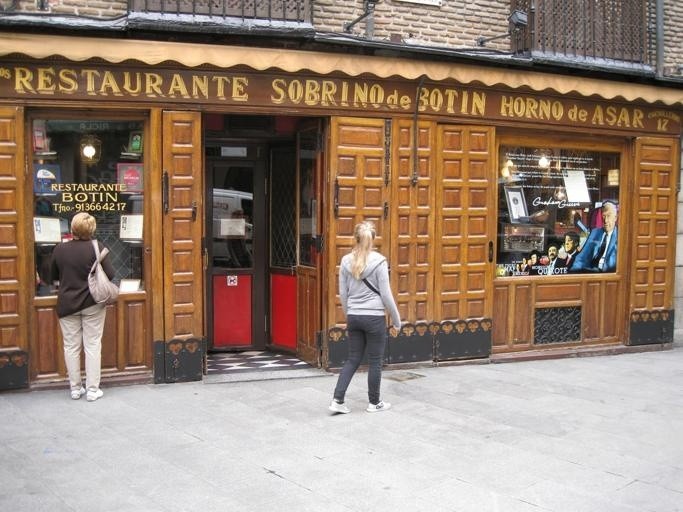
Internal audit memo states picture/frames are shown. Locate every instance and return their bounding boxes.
[127,130,143,154]
[503,186,529,223]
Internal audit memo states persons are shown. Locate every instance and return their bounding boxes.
[47,213,114,401]
[571,199,617,273]
[225,207,251,269]
[514,246,563,274]
[328,221,401,415]
[565,229,579,268]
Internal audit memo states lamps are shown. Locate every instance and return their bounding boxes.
[78,120,102,167]
[477,10,528,46]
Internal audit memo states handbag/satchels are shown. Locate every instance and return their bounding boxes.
[88,263,119,306]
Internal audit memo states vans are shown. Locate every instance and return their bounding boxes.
[210,188,253,267]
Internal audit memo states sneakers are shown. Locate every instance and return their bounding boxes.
[71,387,85,400]
[366,400,392,412]
[328,401,351,413]
[87,389,103,402]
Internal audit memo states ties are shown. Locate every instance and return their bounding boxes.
[591,233,608,268]
